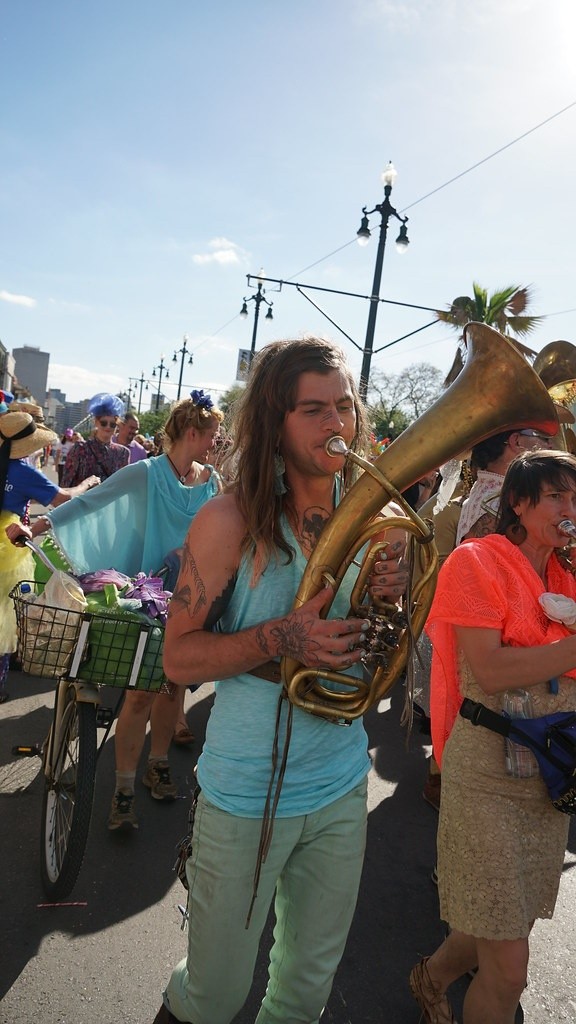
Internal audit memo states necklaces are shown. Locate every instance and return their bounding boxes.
[167,453,191,485]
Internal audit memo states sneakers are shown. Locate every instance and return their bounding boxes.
[142,757,180,800]
[106,786,139,831]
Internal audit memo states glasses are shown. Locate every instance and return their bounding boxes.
[96,418,119,429]
[519,428,556,444]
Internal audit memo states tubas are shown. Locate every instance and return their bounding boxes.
[281,321,574,728]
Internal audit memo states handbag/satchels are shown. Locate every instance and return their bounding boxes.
[460,699,575,817]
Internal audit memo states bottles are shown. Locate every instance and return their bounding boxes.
[501,687,539,777]
[15,584,37,626]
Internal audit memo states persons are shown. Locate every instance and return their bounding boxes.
[172,425,235,743]
[5,387,226,832]
[161,335,409,1024]
[406,450,576,1024]
[0,390,165,702]
[399,426,558,883]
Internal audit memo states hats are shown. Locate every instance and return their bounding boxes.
[7,401,53,431]
[0,411,58,459]
[66,428,74,437]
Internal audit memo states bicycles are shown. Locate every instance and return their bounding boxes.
[8,535,175,902]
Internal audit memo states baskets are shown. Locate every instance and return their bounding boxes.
[8,577,178,693]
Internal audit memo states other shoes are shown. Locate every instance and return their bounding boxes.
[423,777,440,809]
[419,718,433,735]
[172,727,195,743]
[409,956,457,1024]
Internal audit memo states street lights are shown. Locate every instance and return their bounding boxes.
[122,381,136,414]
[354,159,411,404]
[173,333,197,405]
[133,367,148,415]
[388,420,395,437]
[239,267,274,386]
[150,350,170,418]
